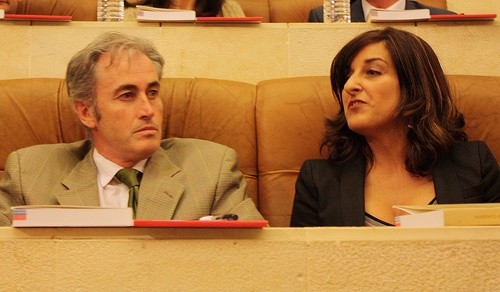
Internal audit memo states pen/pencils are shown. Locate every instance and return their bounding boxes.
[192,213,239,221]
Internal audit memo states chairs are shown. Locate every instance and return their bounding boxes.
[254,74,500,228]
[0,77,257,227]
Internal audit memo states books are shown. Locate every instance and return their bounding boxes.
[8,203,135,229]
[392,202,499,227]
[136,5,199,23]
[365,8,431,23]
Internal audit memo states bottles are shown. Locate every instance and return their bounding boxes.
[97,0,124,21]
[323,0,350,23]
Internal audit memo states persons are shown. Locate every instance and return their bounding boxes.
[0,30,268,227]
[121,0,245,23]
[0,0,98,22]
[307,1,456,23]
[289,29,500,228]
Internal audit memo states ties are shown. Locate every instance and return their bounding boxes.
[116,168,140,220]
